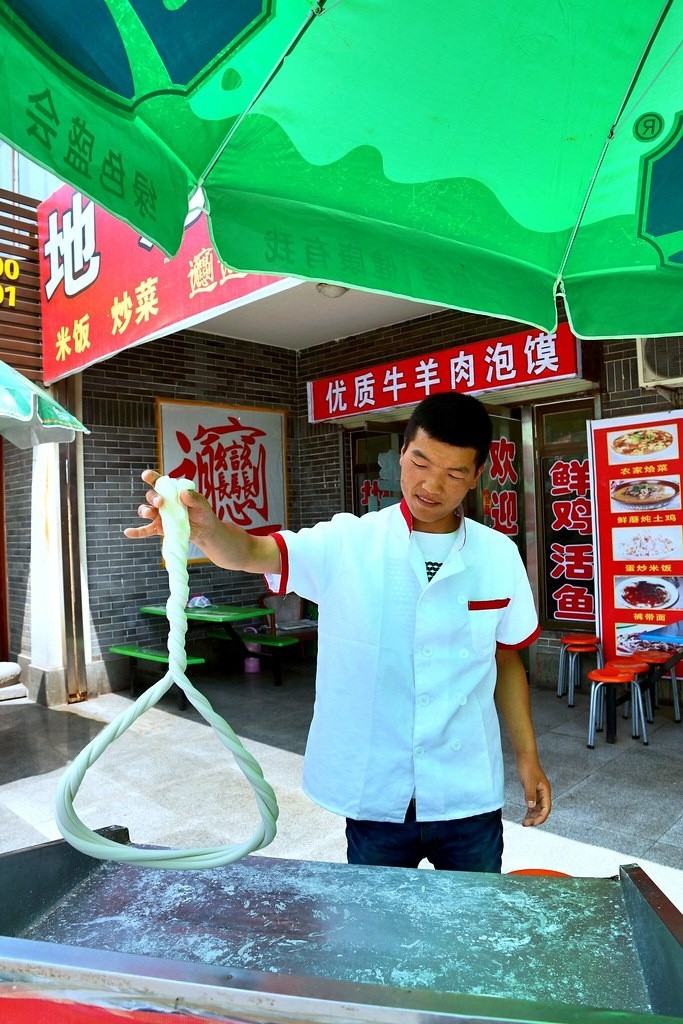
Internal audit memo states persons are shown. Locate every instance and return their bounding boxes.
[120,392,552,874]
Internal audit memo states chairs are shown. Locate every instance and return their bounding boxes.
[257,592,317,674]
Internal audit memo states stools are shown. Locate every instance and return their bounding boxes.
[622,651,682,724]
[562,645,600,697]
[557,634,604,700]
[586,668,648,751]
[596,658,649,740]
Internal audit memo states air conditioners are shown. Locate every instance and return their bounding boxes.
[635,336,683,392]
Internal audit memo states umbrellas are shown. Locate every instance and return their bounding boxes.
[0,358,94,451]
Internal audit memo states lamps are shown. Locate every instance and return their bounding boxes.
[316,283,350,298]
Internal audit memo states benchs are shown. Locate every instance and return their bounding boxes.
[204,629,299,687]
[109,644,205,712]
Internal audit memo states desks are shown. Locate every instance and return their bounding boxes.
[139,605,275,672]
